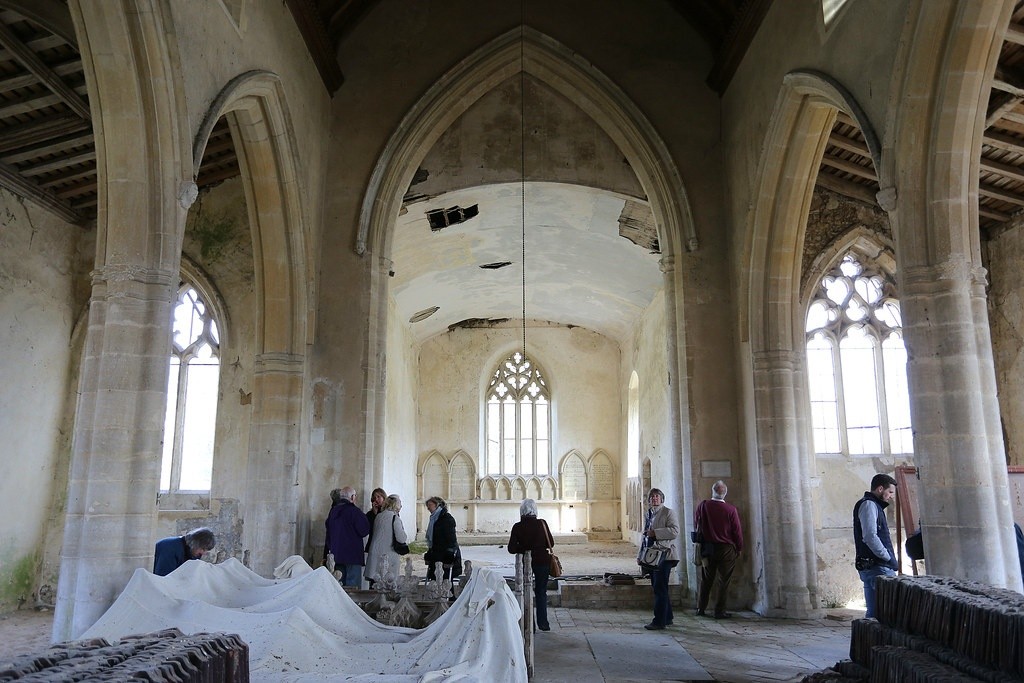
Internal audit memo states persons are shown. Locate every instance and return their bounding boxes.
[694,480,744,619]
[853,474,898,618]
[637,488,680,630]
[508,499,555,634]
[153,529,216,577]
[424,497,462,601]
[323,487,407,590]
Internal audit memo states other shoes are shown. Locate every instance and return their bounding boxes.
[697,608,704,615]
[715,613,731,619]
[644,624,665,630]
[665,620,673,624]
[539,624,550,631]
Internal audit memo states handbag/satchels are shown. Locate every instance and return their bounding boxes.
[639,547,666,569]
[548,553,562,577]
[691,515,702,566]
[392,515,410,555]
